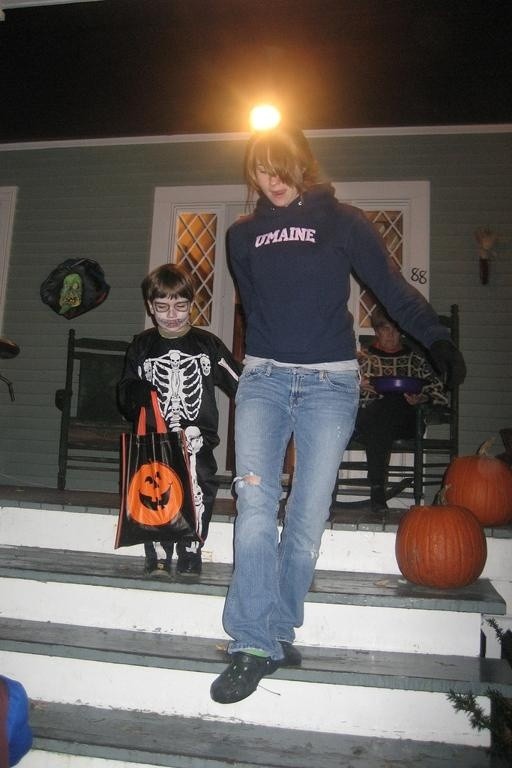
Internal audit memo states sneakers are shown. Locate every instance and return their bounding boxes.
[144,557,171,579]
[370,485,388,513]
[176,556,202,576]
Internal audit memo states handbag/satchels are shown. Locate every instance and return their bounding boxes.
[112,389,202,548]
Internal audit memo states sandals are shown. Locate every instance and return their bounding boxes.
[278,640,302,666]
[209,650,278,705]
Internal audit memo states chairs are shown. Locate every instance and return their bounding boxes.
[54,329,140,495]
[335,303,460,509]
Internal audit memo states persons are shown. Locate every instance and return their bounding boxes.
[355,301,449,512]
[116,264,244,574]
[210,122,466,704]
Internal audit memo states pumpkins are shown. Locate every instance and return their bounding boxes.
[394,483,488,589]
[443,435,512,527]
